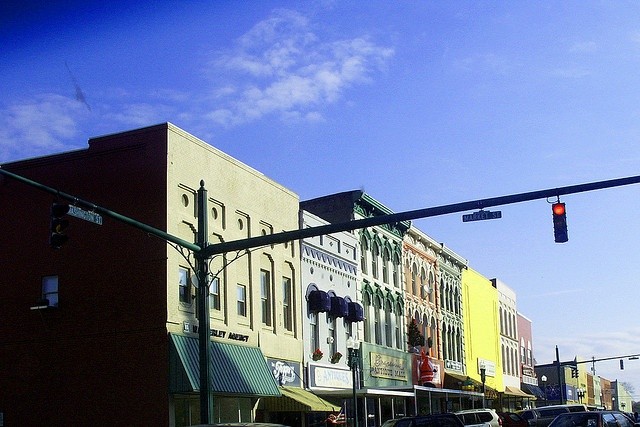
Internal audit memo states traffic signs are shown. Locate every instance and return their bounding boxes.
[463,210,502,221]
[66,202,102,225]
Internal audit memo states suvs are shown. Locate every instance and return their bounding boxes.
[451,407,502,426]
[379,413,466,426]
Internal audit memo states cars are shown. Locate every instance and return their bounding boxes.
[547,410,640,426]
[498,411,528,426]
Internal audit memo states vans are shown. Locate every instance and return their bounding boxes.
[517,404,590,426]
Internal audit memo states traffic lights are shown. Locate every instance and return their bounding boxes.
[575,369,579,378]
[571,368,575,378]
[552,202,568,242]
[49,201,70,247]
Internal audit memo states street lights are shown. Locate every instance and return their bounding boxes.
[621,401,627,410]
[480,362,486,393]
[600,392,603,406]
[345,335,361,427]
[612,397,615,407]
[577,388,586,403]
[542,374,548,405]
